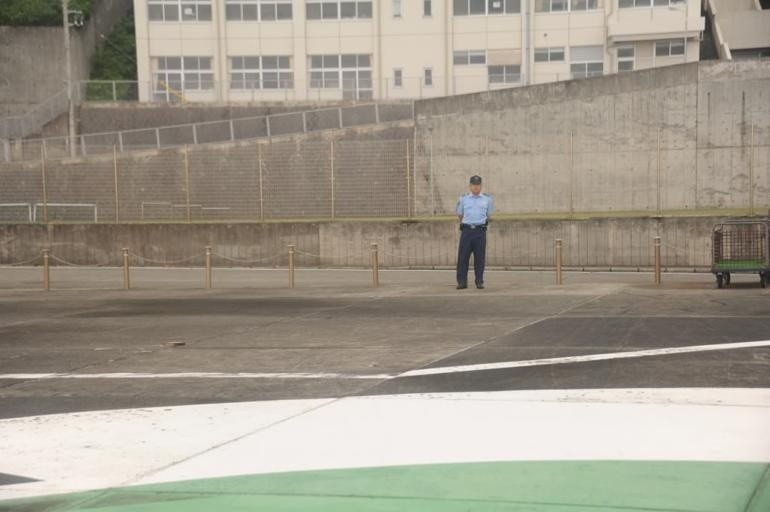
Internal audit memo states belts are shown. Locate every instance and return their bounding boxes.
[462,225,486,230]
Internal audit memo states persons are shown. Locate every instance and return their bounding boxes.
[451,173,494,292]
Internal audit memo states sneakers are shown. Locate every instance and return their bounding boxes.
[475,279,485,288]
[457,282,468,289]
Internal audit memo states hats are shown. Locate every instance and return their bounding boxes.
[470,176,481,184]
